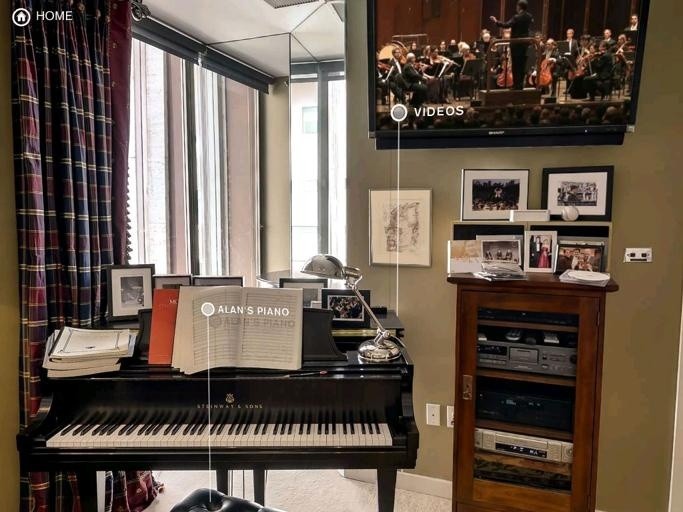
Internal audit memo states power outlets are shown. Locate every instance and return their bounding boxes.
[446,405,454,428]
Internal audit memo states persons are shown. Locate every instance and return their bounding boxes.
[531,236,550,268]
[486,249,512,261]
[557,182,597,206]
[375,0,638,110]
[559,248,599,272]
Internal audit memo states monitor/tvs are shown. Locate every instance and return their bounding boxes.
[366,0,652,151]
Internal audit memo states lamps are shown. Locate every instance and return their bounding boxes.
[299,253,406,363]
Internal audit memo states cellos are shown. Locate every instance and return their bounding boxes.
[497,50,513,87]
[535,42,556,87]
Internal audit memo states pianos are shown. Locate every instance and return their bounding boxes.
[15,306,417,511]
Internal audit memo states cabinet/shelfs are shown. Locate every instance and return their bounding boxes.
[446,273,618,512]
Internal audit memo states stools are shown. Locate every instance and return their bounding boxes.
[168,487,287,512]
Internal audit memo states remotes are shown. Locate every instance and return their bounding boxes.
[478,333,488,341]
[505,329,522,341]
[543,333,560,344]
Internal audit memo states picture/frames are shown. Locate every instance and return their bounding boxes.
[105,264,155,322]
[459,168,529,221]
[475,234,523,266]
[368,188,431,269]
[541,165,614,221]
[523,230,557,273]
[558,244,604,275]
[321,289,370,329]
[152,276,190,288]
[279,278,327,309]
[192,277,242,286]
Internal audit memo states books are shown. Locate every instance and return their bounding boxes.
[43,325,135,379]
[148,285,304,375]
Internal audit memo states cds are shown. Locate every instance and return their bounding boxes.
[568,270,610,281]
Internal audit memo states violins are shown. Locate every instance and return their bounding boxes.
[584,50,605,62]
[378,53,477,72]
